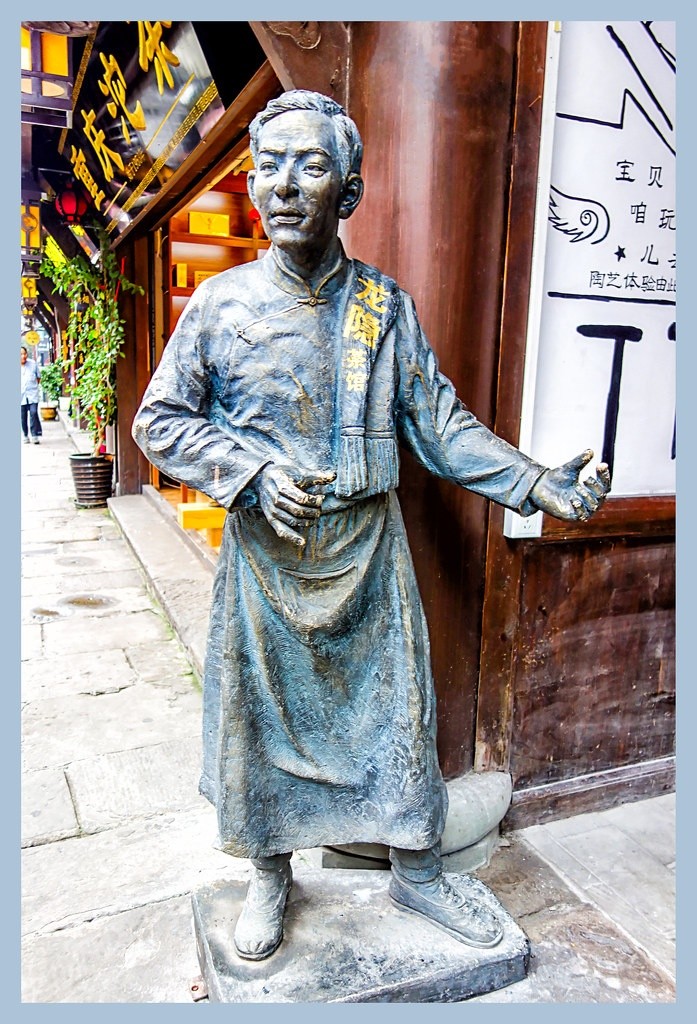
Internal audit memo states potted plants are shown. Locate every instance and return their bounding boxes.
[38,219,147,504]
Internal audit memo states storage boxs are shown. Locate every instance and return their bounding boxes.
[188,212,230,236]
[173,263,188,288]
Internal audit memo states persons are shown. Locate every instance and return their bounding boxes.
[132,89,610,958]
[21,346,44,444]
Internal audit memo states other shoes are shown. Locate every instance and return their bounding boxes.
[33,437,39,444]
[25,437,31,444]
